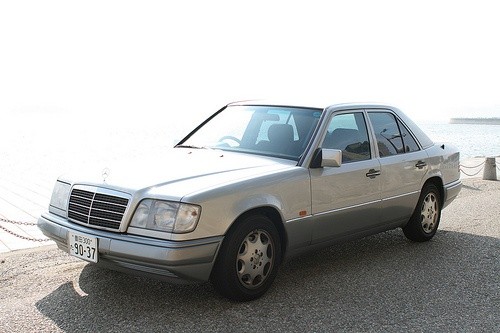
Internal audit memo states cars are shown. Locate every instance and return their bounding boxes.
[37,96,462,301]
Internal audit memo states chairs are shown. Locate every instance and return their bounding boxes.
[256,123,301,158]
